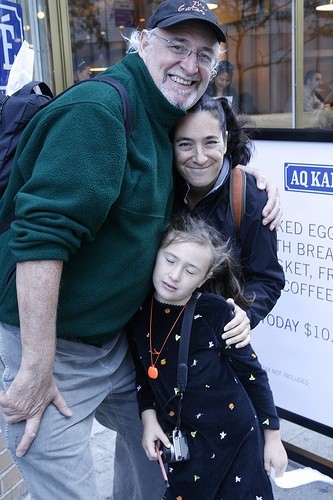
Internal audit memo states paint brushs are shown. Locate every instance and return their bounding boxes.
[154,443,171,489]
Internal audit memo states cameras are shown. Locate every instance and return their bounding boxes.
[161,430,190,462]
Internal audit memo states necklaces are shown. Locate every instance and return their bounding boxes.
[148,298,186,380]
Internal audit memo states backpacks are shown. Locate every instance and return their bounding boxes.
[0,76,132,235]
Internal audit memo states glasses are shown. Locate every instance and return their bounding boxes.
[150,32,217,69]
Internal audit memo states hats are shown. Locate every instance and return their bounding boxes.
[147,0,226,44]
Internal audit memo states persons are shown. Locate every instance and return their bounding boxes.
[283,70,325,111]
[205,60,238,112]
[172,93,286,348]
[127,215,288,500]
[72,56,90,84]
[0,0,282,500]
